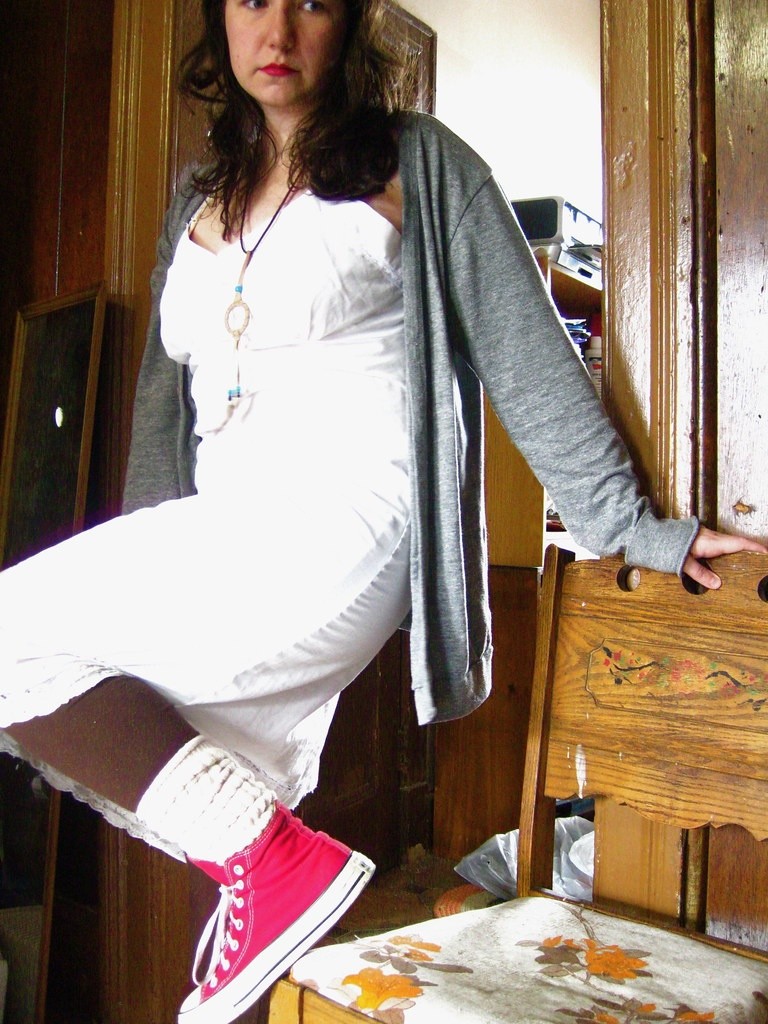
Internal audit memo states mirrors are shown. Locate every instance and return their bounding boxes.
[0,279,108,1024]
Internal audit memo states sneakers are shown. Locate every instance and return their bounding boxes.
[179,799,377,1024]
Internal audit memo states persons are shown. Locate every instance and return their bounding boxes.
[0,1,767,1024]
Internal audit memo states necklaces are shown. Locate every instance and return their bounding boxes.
[225,158,305,400]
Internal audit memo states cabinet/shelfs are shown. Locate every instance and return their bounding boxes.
[483,254,603,567]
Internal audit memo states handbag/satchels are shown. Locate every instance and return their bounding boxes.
[454,814,594,903]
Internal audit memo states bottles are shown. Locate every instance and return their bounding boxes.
[583,336,602,394]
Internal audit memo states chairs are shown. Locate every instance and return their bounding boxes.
[255,544,768,1024]
